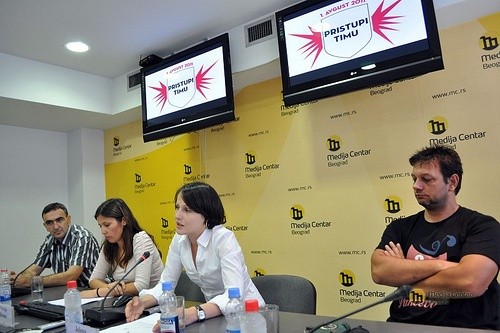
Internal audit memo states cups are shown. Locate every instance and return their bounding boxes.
[259,303,280,333]
[31,276,43,303]
[175,296,186,332]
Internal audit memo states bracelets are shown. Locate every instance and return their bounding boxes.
[96,288,100,297]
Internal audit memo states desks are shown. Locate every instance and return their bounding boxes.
[0,286,500,333]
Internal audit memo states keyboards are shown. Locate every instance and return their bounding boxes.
[17,300,65,321]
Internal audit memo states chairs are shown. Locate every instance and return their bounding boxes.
[250,276,316,315]
[174,271,206,303]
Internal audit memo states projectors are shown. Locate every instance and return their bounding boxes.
[139,54,164,67]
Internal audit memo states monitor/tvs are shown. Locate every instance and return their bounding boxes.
[140,30,236,143]
[275,0,444,107]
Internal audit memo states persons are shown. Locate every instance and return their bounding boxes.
[371,146,500,331]
[125,182,266,333]
[7,202,100,289]
[79,198,164,298]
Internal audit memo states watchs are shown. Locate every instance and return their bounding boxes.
[194,304,206,322]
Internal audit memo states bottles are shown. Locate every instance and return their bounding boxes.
[240,299,267,333]
[224,287,244,333]
[158,282,179,333]
[0,267,12,305]
[64,281,83,333]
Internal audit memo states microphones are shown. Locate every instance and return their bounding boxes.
[9,248,53,298]
[86,250,151,325]
[304,284,413,333]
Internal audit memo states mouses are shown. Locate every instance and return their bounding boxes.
[112,292,134,307]
